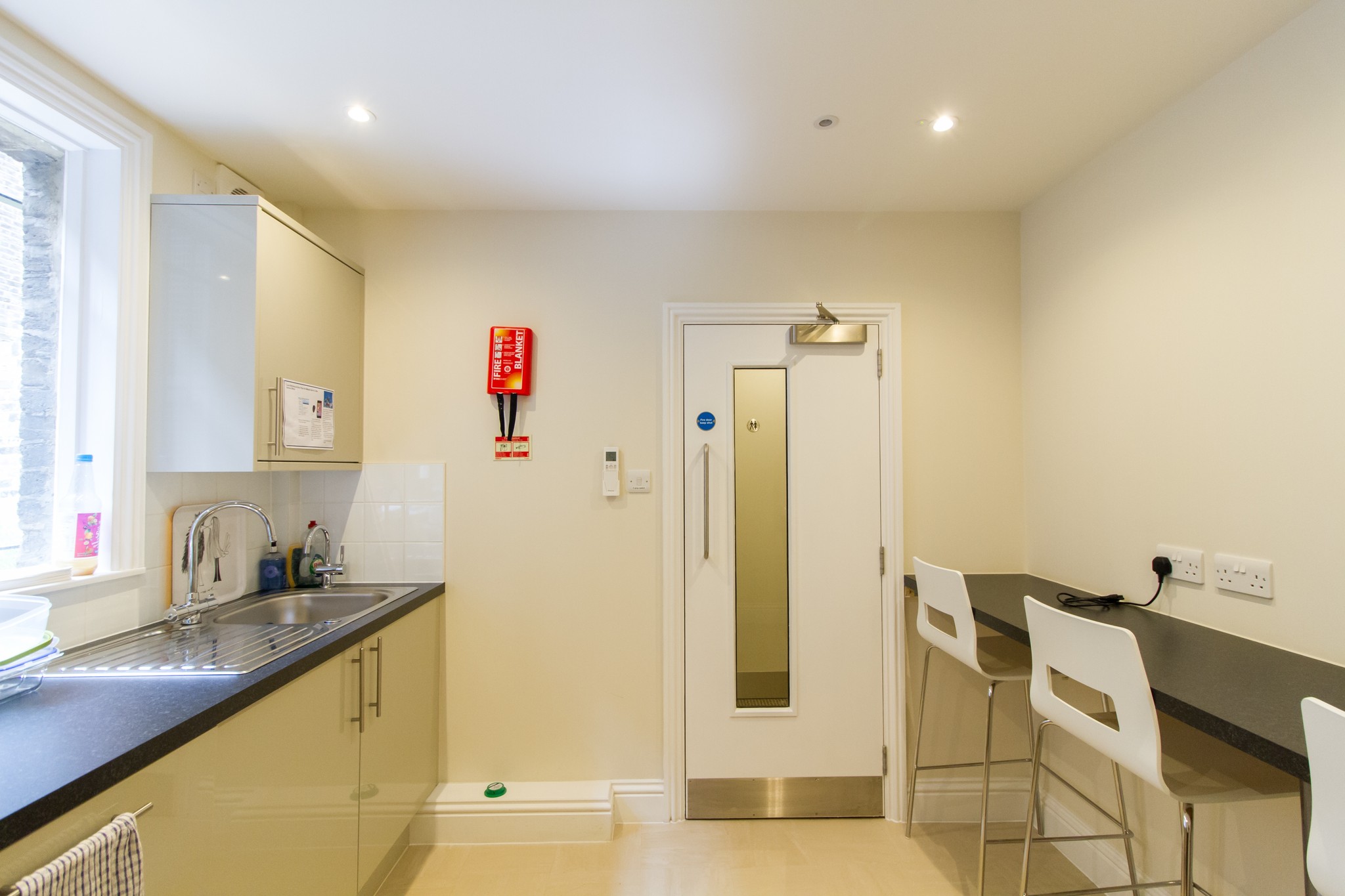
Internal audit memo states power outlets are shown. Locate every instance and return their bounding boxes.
[1157,544,1205,585]
[1214,555,1274,599]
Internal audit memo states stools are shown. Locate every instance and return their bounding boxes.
[906,556,1138,896]
[1021,594,1302,896]
[1301,697,1345,896]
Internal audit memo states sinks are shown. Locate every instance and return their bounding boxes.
[210,583,418,625]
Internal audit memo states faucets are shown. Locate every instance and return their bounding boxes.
[302,524,346,588]
[163,499,279,629]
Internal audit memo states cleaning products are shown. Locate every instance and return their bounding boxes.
[259,541,287,589]
[298,520,322,588]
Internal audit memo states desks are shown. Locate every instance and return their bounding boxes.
[904,573,1344,784]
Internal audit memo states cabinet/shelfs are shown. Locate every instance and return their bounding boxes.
[219,595,443,896]
[149,195,365,473]
